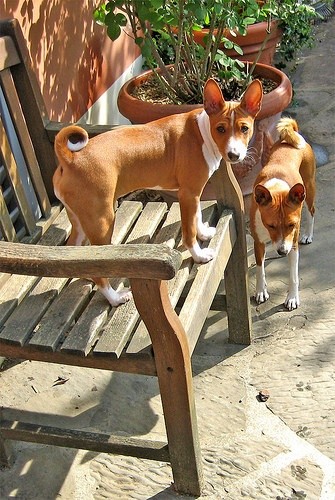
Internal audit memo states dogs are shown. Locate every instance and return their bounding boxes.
[52,77,264,308]
[251,116,314,311]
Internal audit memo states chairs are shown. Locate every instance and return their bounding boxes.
[0,17,251,497]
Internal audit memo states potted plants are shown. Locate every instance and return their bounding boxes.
[163,0,303,107]
[91,0,324,222]
